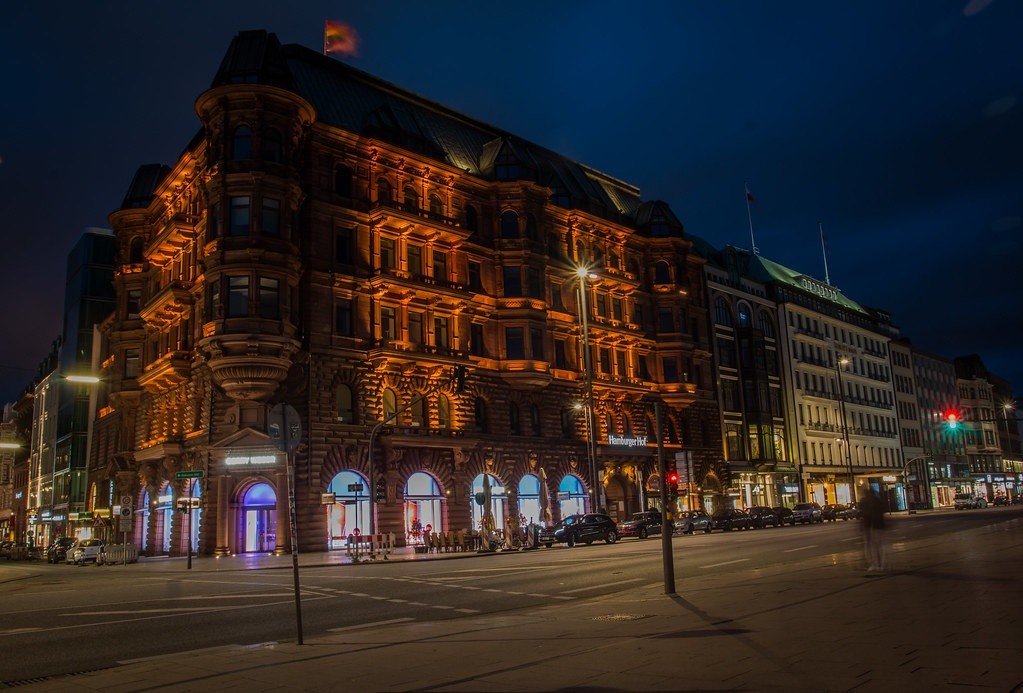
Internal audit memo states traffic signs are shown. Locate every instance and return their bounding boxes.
[348,484,363,492]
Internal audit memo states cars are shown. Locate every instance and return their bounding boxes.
[65,538,105,565]
[47,537,79,564]
[616,511,673,540]
[0,540,30,560]
[792,503,863,524]
[953,494,1023,510]
[672,510,713,535]
[745,506,778,530]
[772,507,796,527]
[712,508,753,532]
[538,513,618,546]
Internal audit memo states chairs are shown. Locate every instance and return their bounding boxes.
[424,531,466,554]
[345,534,395,556]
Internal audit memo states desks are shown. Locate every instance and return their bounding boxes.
[439,536,482,551]
[349,535,390,552]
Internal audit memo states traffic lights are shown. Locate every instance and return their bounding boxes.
[457,366,470,393]
[668,474,679,501]
[949,414,956,429]
[178,500,187,514]
[372,480,386,503]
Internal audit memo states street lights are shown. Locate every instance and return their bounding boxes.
[577,267,601,514]
[838,359,856,502]
[1003,405,1017,494]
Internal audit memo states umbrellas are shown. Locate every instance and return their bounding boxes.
[481,473,496,540]
[536,468,552,523]
